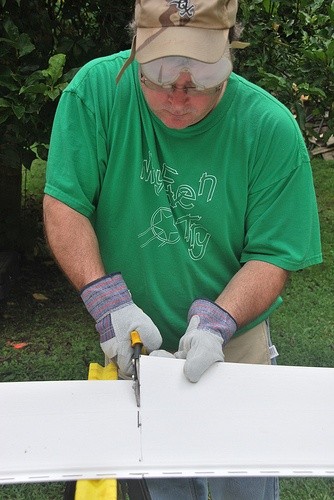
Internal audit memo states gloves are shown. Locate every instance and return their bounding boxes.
[79,271,162,377]
[174,298,238,383]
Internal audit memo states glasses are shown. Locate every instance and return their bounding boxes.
[139,72,224,96]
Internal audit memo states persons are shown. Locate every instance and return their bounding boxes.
[42,0,324,500]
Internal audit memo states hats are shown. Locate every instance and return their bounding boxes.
[131,0,238,64]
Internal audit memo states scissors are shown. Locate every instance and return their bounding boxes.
[130,330,145,406]
